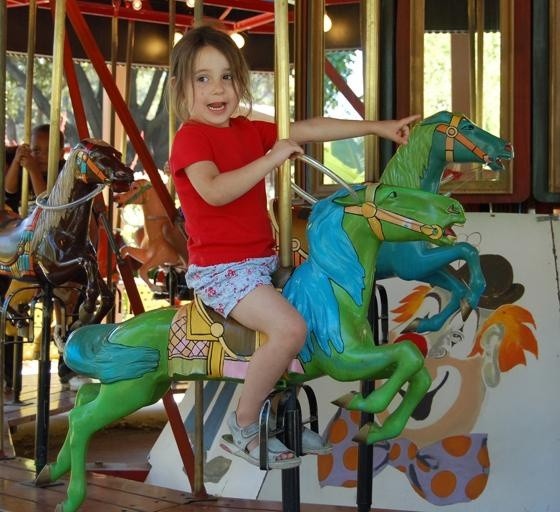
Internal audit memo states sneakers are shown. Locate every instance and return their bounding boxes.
[62,376,92,390]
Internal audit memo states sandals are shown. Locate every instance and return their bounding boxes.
[268,413,334,455]
[220,411,302,469]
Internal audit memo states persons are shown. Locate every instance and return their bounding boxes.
[161,26,422,471]
[1,143,102,405]
[5,125,75,208]
